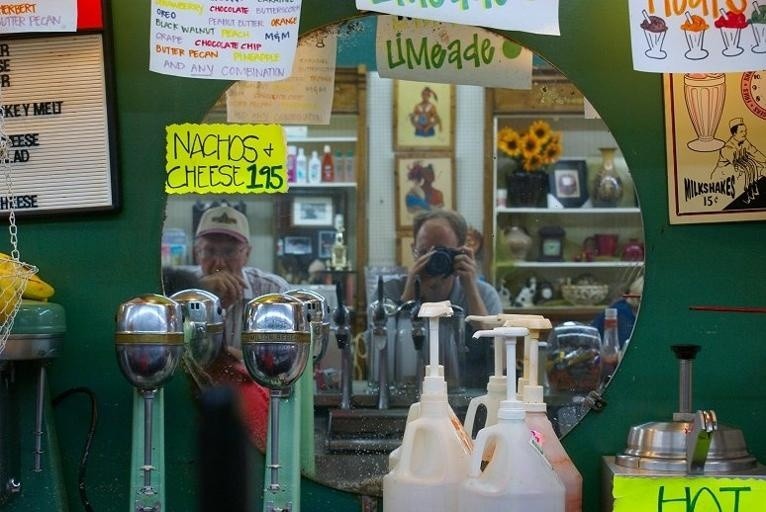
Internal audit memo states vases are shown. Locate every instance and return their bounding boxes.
[591,148,623,209]
[504,171,550,208]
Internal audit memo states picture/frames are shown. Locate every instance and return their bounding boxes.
[397,230,417,270]
[0,1,124,225]
[395,151,457,230]
[394,76,455,152]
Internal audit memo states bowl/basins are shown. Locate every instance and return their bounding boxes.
[559,286,607,306]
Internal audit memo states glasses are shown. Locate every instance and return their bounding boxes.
[198,248,243,258]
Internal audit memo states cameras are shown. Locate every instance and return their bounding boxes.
[423,246,465,279]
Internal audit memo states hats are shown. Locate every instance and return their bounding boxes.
[195,206,250,244]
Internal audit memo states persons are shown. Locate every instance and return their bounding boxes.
[592,278,648,381]
[712,117,766,200]
[170,203,290,349]
[407,162,444,213]
[409,88,442,136]
[370,212,503,390]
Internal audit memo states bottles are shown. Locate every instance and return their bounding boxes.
[583,234,619,261]
[282,142,356,189]
[602,308,620,380]
[330,233,347,272]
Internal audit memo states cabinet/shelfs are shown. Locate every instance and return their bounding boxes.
[491,114,644,315]
[286,136,358,188]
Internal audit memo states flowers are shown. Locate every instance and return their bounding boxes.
[495,122,563,170]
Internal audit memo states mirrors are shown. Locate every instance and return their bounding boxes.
[162,15,646,499]
